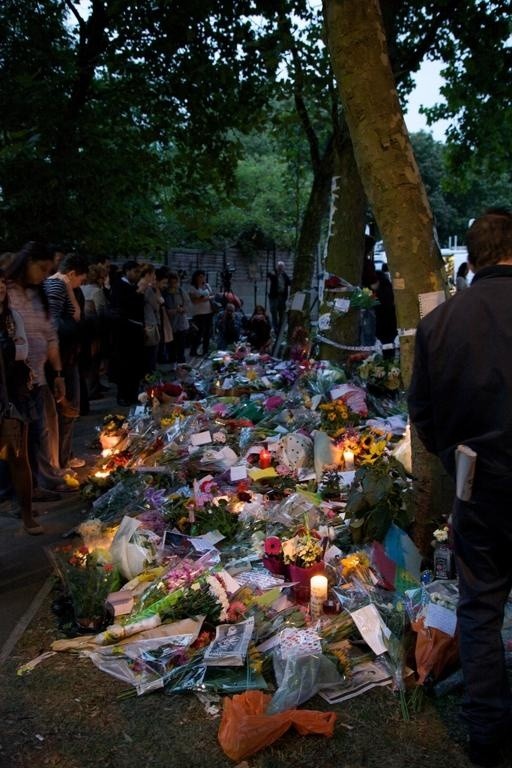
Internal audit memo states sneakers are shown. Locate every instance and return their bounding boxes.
[52,480,79,493]
[69,458,85,466]
[58,468,75,476]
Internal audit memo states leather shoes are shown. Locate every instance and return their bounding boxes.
[30,488,63,502]
[22,521,44,535]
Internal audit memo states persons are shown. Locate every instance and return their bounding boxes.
[44,253,88,492]
[0,241,62,520]
[264,261,291,335]
[51,245,91,417]
[187,271,216,356]
[0,268,43,536]
[246,305,271,351]
[360,236,374,347]
[94,251,112,376]
[163,273,189,365]
[457,263,469,293]
[217,292,243,311]
[113,261,148,407]
[136,263,160,379]
[216,305,243,346]
[77,265,112,400]
[407,206,512,768]
[374,271,398,364]
[152,269,172,362]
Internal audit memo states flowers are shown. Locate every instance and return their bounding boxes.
[279,531,324,563]
[126,545,217,614]
[159,410,186,424]
[349,347,404,393]
[330,580,415,726]
[89,409,131,435]
[324,276,342,289]
[115,637,267,705]
[403,570,462,719]
[50,574,230,654]
[343,428,410,544]
[319,398,349,429]
[50,613,215,690]
[63,487,169,537]
[217,609,356,762]
[41,543,121,619]
[310,285,382,342]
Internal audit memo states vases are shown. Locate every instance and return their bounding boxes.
[98,435,123,450]
[69,614,103,639]
[287,560,323,607]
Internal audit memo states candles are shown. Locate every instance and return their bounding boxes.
[308,571,329,624]
[342,448,358,475]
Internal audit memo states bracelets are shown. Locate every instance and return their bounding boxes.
[52,370,65,377]
